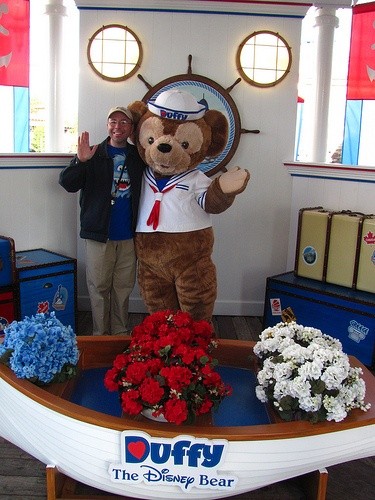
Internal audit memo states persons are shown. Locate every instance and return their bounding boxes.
[58,105,149,336]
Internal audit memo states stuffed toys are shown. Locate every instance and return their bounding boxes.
[125,89,249,326]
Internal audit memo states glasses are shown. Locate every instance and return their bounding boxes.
[110,119,129,126]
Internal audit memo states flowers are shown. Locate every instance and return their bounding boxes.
[252,320,371,425]
[0,311,80,387]
[105,308,232,427]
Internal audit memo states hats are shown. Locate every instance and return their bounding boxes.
[107,107,134,123]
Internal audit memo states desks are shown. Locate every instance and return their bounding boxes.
[0,327,375,500]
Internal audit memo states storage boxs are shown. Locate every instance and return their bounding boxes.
[0,235,77,331]
[262,207,375,374]
